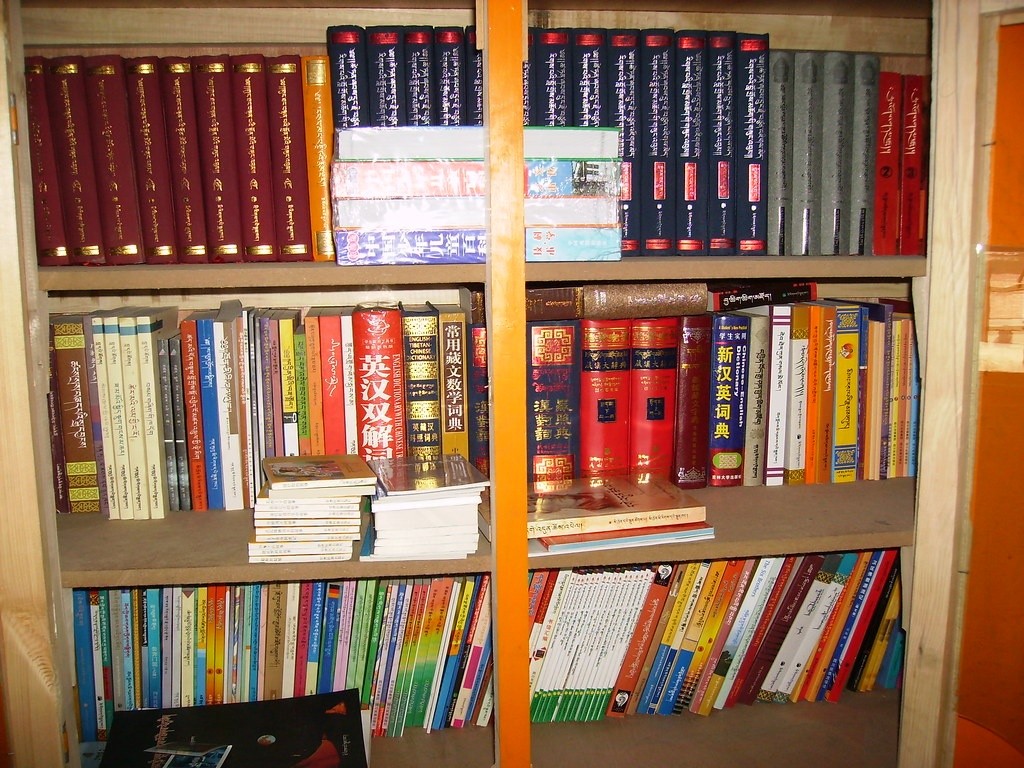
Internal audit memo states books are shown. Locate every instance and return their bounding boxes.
[458,282,921,558]
[23,24,484,266]
[472,551,907,728]
[65,575,493,768]
[331,28,931,262]
[50,291,483,564]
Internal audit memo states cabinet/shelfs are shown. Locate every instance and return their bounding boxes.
[0,0,996,768]
